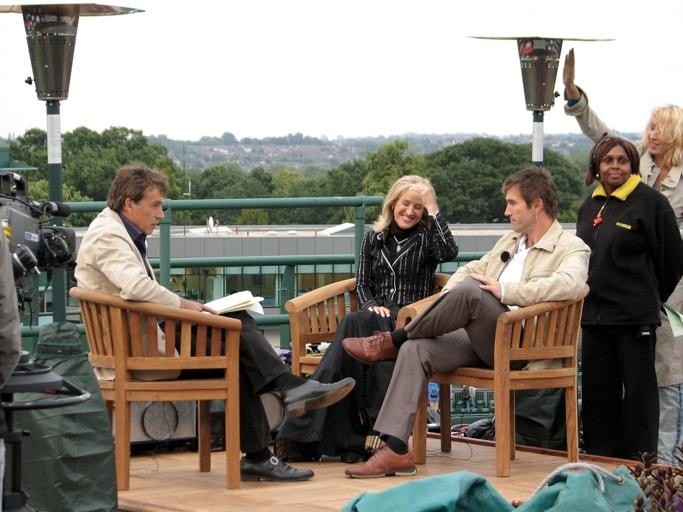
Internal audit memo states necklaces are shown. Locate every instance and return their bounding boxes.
[392,234,409,253]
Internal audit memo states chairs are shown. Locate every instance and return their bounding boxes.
[68,286,243,491]
[284,272,591,477]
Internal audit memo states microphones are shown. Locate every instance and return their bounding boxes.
[377,239,383,249]
[500,251,511,262]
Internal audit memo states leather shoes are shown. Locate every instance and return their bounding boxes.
[344,443,418,480]
[342,326,401,367]
[240,453,316,483]
[279,377,356,420]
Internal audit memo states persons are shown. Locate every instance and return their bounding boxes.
[272,175,460,462]
[0,219,22,512]
[74,162,357,481]
[575,135,683,466]
[559,47,683,466]
[342,166,592,477]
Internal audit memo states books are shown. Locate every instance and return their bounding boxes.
[199,289,264,316]
